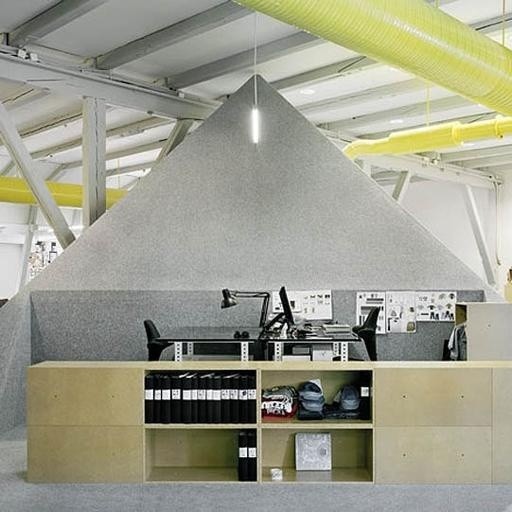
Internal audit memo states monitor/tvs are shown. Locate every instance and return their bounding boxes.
[279,285,294,324]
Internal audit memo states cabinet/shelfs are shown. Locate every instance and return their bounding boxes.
[454,301,511,360]
[30,359,144,484]
[144,360,374,485]
[375,360,510,484]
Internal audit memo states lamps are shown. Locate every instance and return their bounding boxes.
[222,289,270,327]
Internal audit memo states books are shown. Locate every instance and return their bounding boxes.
[238,431,257,481]
[145,372,256,423]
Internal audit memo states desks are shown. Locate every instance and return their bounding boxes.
[156,320,358,364]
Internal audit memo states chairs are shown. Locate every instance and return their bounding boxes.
[145,318,173,360]
[354,303,381,361]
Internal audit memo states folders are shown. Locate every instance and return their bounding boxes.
[144,372,257,423]
[238,429,257,481]
